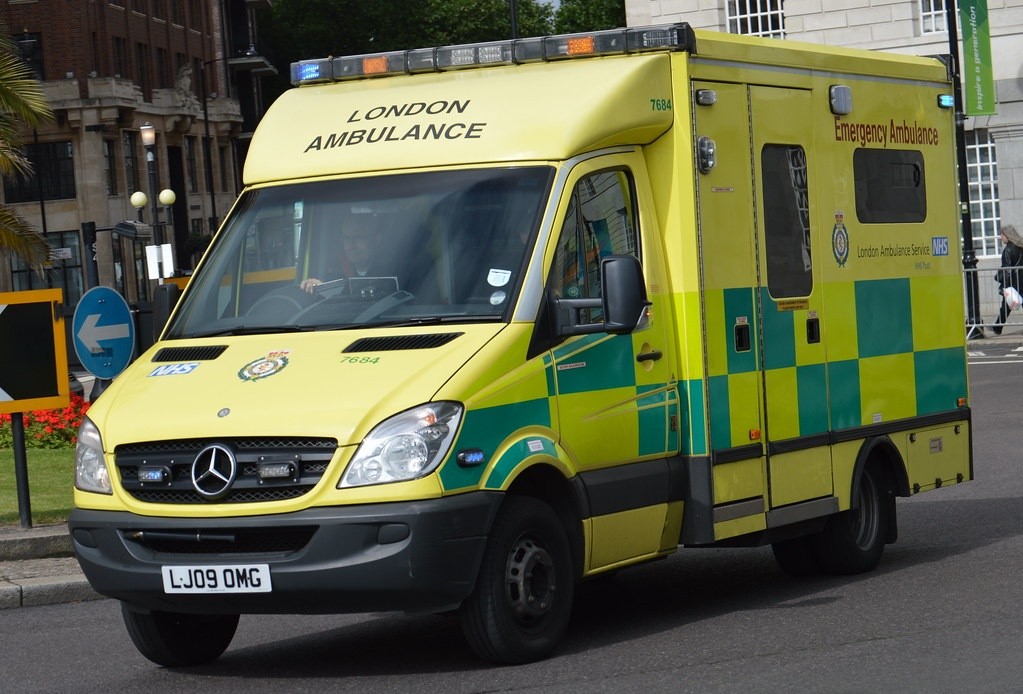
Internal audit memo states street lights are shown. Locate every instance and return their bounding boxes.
[140,122,161,245]
[130,190,147,302]
[158,188,178,278]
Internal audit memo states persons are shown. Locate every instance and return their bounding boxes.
[298,223,395,295]
[986,223,1022,334]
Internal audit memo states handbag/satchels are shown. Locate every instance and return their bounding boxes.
[994,268,1013,284]
[1003,286,1023,311]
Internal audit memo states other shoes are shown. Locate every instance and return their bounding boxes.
[986,323,1000,335]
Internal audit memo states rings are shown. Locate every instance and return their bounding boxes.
[313,284,317,286]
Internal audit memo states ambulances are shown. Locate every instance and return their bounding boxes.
[68,22,976,670]
